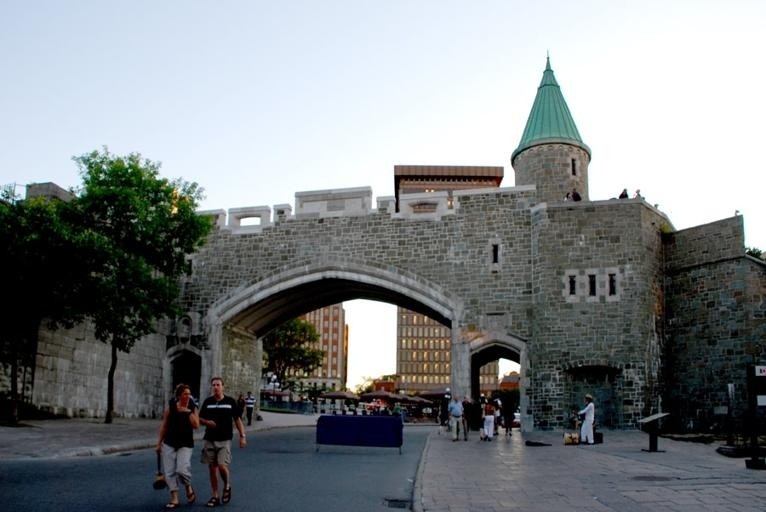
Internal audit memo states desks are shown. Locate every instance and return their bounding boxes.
[316,415,404,454]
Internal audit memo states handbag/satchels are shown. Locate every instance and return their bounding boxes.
[153,470,167,490]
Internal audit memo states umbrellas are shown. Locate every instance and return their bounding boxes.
[319,390,434,403]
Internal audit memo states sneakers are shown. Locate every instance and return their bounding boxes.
[493,432,512,436]
[453,437,467,442]
[480,435,491,442]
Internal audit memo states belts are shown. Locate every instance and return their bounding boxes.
[453,415,462,418]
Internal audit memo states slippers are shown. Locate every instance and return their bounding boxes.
[186,488,197,503]
[165,502,180,509]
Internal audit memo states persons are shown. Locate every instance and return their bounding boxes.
[448,393,515,441]
[199,377,246,508]
[237,391,256,425]
[165,383,199,411]
[576,393,595,444]
[156,385,200,508]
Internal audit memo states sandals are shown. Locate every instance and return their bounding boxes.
[206,496,220,507]
[222,487,231,503]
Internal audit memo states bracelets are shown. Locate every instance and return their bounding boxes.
[240,434,245,437]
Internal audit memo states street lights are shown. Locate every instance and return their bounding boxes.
[444,387,452,424]
[268,374,279,400]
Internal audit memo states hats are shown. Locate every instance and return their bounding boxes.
[585,393,593,399]
